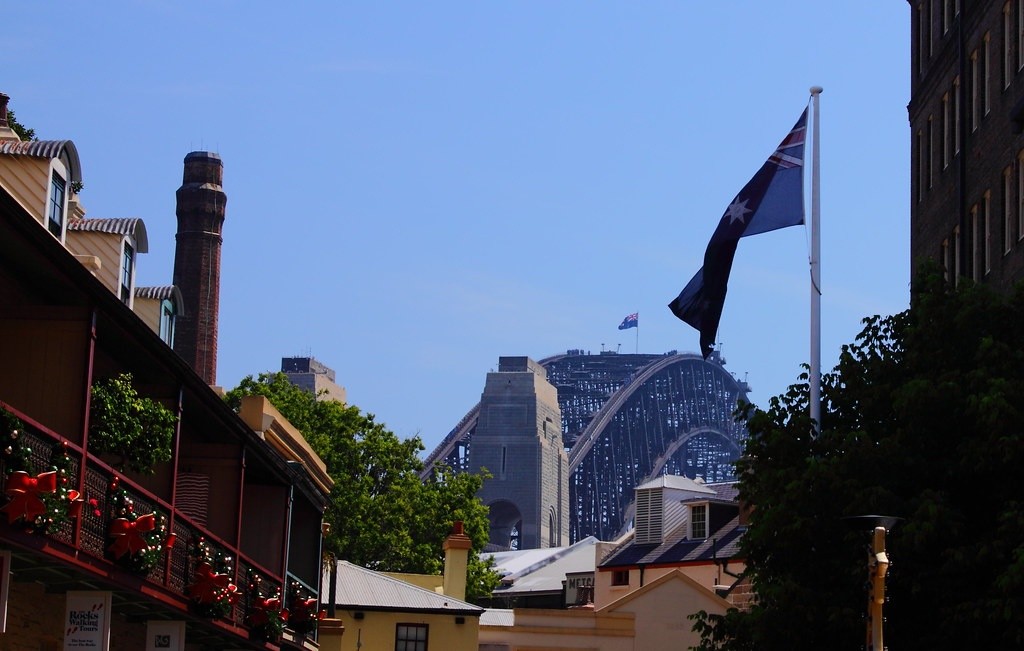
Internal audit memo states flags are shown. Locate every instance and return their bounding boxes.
[667,106,805,360]
[618,314,638,330]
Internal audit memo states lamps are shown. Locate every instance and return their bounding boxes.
[455,617,464,624]
[355,612,363,618]
